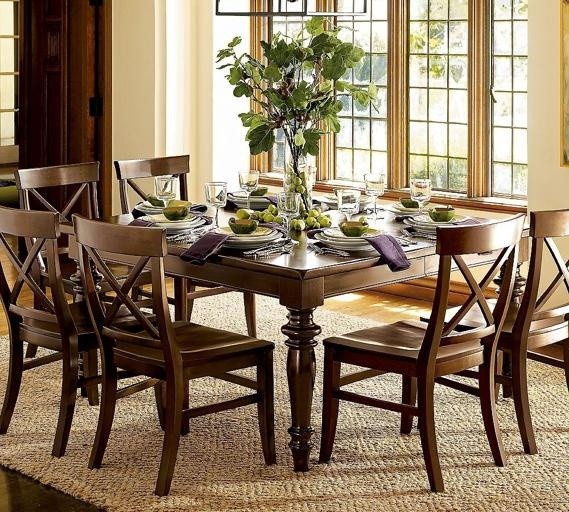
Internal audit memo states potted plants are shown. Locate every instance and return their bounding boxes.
[217,15,379,216]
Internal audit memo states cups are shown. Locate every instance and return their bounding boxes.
[237,168,259,210]
[203,181,228,229]
[153,176,177,210]
[363,173,385,220]
[277,192,302,250]
[336,189,360,224]
[410,179,432,217]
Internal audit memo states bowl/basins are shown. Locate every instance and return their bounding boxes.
[231,218,258,234]
[163,207,189,220]
[429,207,456,221]
[338,221,369,237]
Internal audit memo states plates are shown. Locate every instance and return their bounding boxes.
[311,192,378,211]
[134,212,207,234]
[313,227,388,251]
[403,215,470,235]
[212,227,283,247]
[135,200,195,215]
[386,202,451,217]
[223,190,275,209]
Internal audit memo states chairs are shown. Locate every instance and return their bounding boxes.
[0,205,167,469]
[15,162,158,397]
[115,155,257,338]
[418,210,569,454]
[71,213,275,495]
[320,212,526,492]
